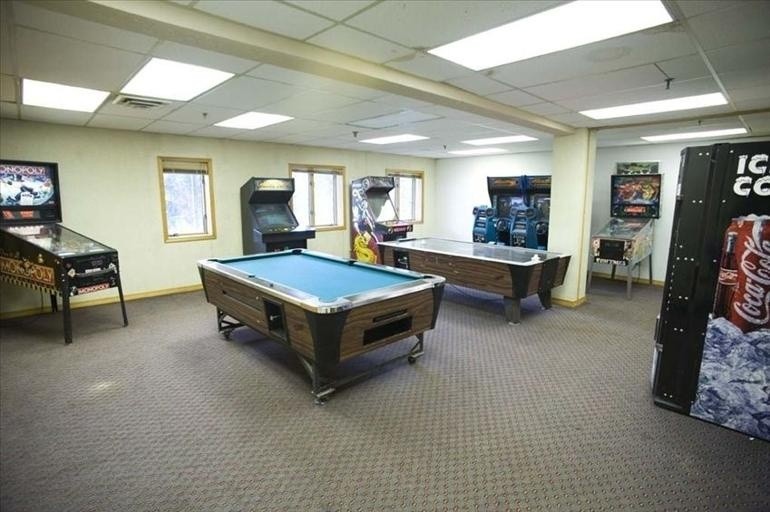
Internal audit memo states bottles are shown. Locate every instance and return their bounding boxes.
[712,231,738,322]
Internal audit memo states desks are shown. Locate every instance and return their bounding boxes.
[377,235,570,326]
[199,248,447,407]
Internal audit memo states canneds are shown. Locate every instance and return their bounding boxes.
[723,218,770,334]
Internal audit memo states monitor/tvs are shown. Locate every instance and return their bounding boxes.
[251,203,297,227]
[495,194,525,219]
[367,192,398,221]
[533,195,550,220]
